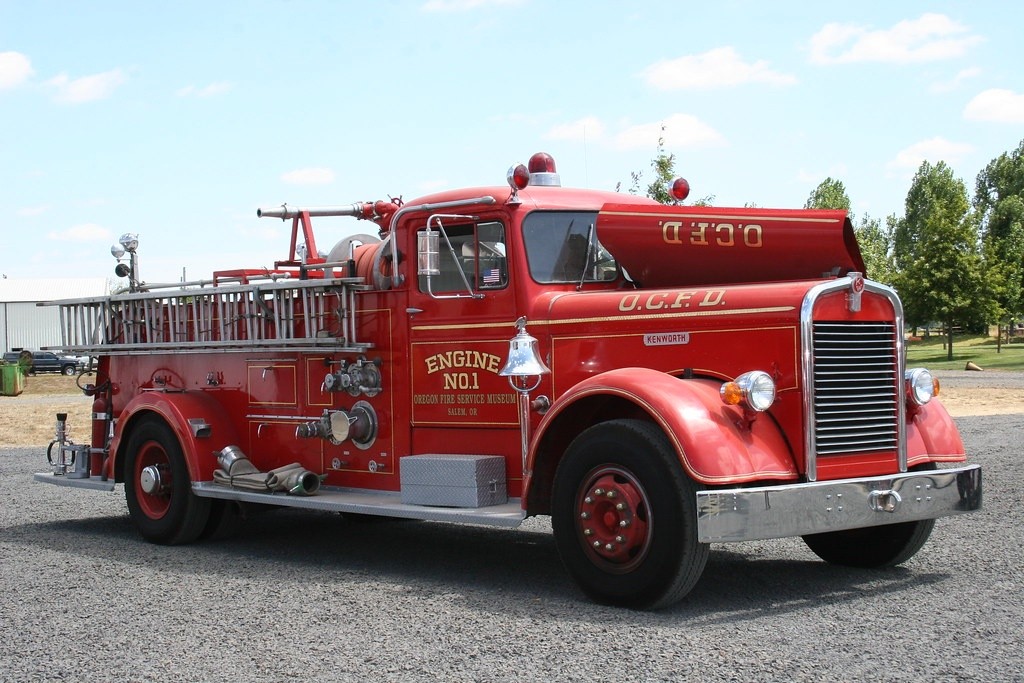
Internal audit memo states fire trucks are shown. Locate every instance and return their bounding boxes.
[35,153,985,613]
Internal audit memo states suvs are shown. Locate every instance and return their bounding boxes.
[2,351,81,375]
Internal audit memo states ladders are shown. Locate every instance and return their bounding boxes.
[32,276,376,356]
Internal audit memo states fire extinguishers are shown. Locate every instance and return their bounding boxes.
[82,382,110,478]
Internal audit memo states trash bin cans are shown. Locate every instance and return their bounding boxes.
[0,358,24,396]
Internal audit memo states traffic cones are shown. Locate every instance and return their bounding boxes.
[965,361,984,372]
